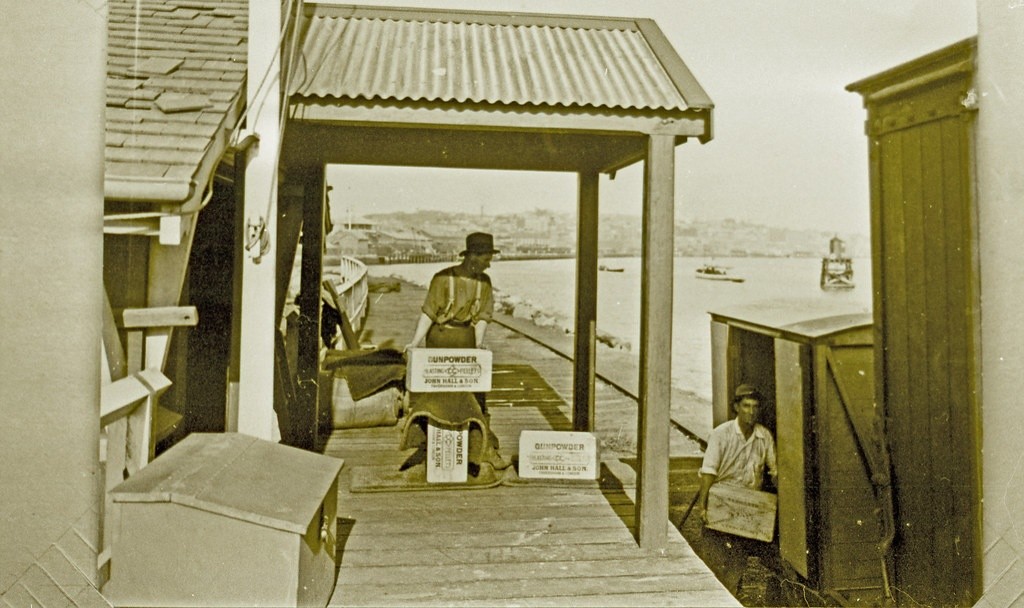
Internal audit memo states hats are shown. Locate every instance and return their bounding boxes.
[459,232,500,256]
[729,384,768,412]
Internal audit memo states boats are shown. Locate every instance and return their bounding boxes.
[695,271,745,284]
[599,264,625,273]
[820,237,855,292]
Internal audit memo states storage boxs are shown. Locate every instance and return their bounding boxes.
[426,416,469,483]
[519,430,601,481]
[704,484,778,543]
[406,347,492,393]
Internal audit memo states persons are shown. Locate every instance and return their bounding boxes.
[698,385,778,598]
[289,233,339,351]
[404,232,512,470]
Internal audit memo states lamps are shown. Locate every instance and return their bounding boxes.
[231,130,261,150]
